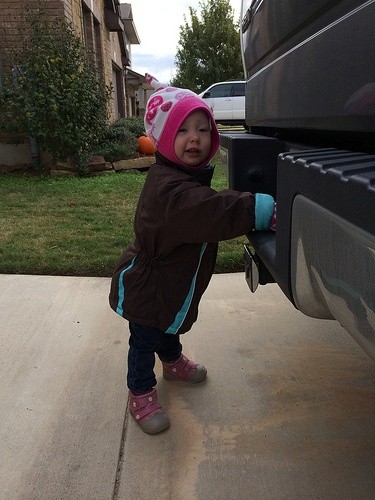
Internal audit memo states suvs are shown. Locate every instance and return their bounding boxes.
[227,0,375,357]
[198,80,247,126]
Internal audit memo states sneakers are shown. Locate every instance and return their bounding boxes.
[130,387,170,435]
[162,354,207,383]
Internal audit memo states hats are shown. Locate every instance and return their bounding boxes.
[144,86,219,169]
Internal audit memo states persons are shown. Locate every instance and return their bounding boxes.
[109,73,277,435]
